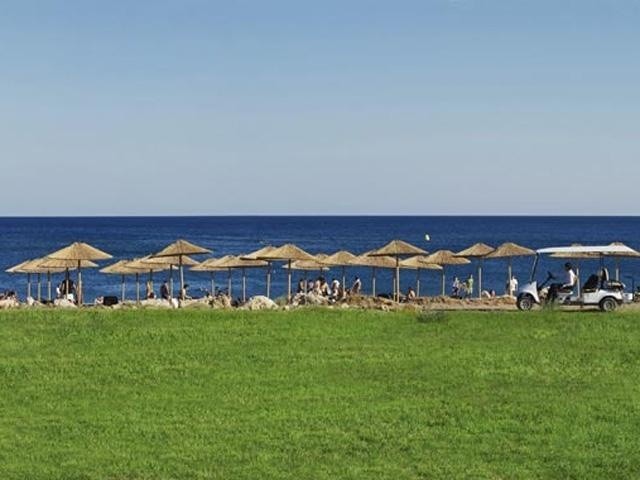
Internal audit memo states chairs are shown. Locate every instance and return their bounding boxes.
[582,274,599,292]
[600,266,622,289]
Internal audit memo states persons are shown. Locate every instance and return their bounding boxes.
[546,262,576,301]
[633,285,640,302]
